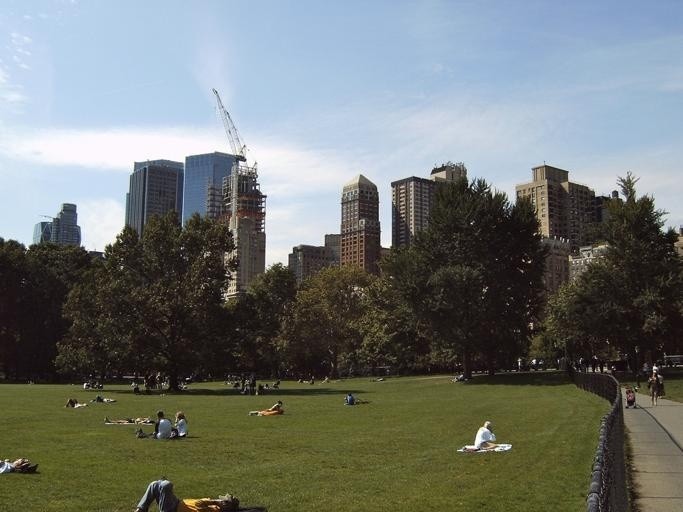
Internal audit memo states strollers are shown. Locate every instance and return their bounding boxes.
[625,384,638,409]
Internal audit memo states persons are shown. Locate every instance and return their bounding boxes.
[132,479,239,511]
[472,419,499,451]
[151,410,171,439]
[88,394,116,403]
[348,393,356,404]
[82,374,329,394]
[647,369,659,406]
[63,398,87,408]
[248,399,283,417]
[170,411,187,437]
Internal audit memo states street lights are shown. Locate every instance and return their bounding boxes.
[605,337,612,370]
[635,344,641,389]
